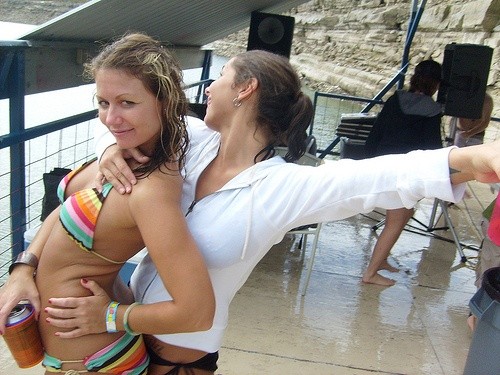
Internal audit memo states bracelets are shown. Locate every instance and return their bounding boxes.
[9,252,38,275]
[106,301,119,334]
[123,302,142,337]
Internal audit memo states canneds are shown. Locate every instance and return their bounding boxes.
[6,305,31,324]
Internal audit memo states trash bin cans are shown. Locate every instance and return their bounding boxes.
[463,266,499,375]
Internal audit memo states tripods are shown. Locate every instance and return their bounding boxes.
[373,115,466,263]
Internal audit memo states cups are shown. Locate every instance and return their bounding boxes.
[3,304,44,368]
[463,266,500,375]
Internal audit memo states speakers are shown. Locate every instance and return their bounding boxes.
[437,44,494,119]
[247,11,295,62]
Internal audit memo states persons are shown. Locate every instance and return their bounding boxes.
[449,93,493,198]
[361,60,444,286]
[0,30,215,375]
[94,49,500,375]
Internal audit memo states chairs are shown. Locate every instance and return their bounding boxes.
[273,146,322,297]
